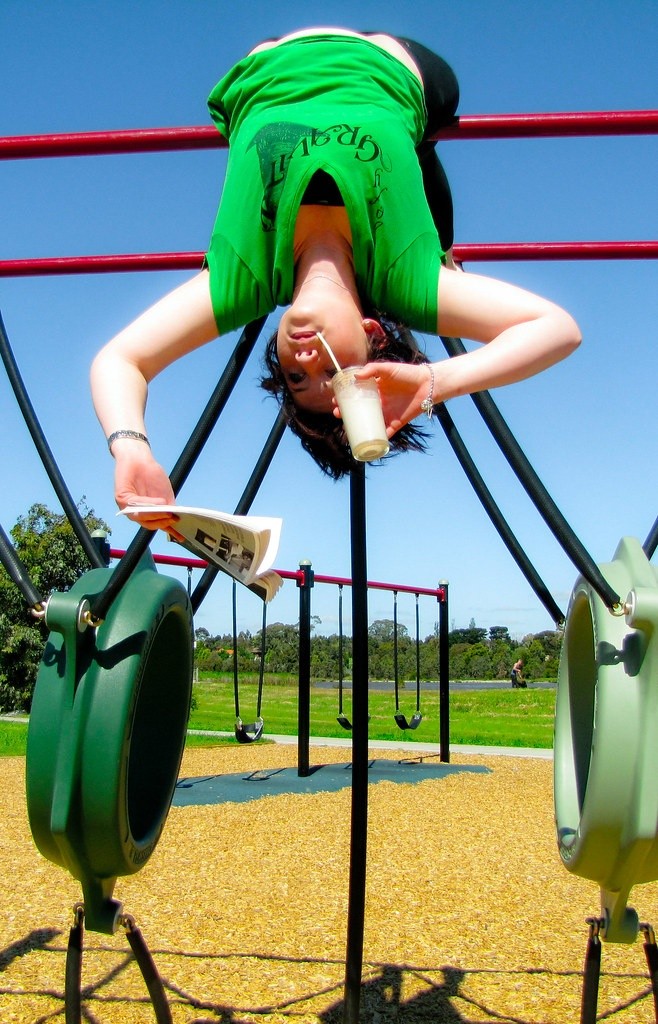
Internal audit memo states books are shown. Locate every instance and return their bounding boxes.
[116,502,283,605]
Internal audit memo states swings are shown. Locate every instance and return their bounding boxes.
[231,579,268,743]
[393,591,423,730]
[336,584,371,730]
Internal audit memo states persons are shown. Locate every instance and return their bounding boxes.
[91,27,582,529]
[420,363,433,418]
[511,659,523,688]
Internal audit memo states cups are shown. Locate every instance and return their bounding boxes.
[332,365,389,462]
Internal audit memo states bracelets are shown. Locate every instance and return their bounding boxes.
[108,430,151,457]
